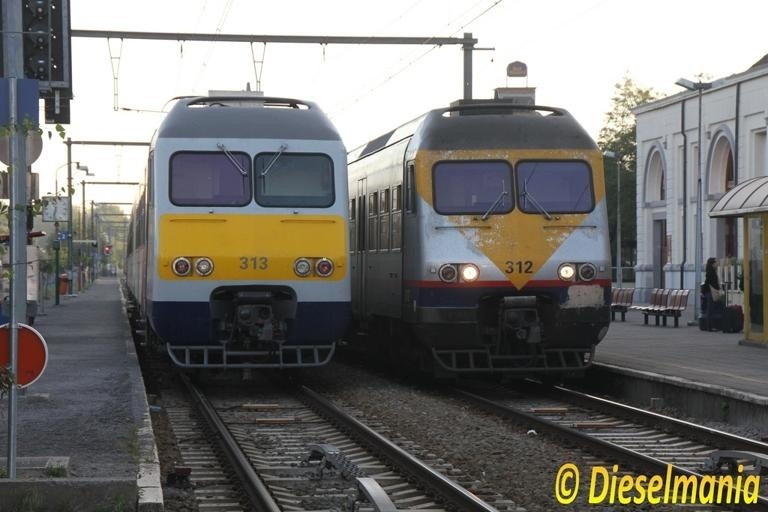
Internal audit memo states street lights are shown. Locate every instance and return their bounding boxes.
[675,77,712,326]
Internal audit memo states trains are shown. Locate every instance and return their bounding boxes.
[123,95,353,388]
[347,104,612,382]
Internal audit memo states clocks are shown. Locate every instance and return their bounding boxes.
[42,196,71,222]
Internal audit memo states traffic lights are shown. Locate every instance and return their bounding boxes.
[105,246,110,254]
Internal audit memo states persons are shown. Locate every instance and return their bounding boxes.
[704,256,727,333]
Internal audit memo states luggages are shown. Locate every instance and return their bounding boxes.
[724,282,744,333]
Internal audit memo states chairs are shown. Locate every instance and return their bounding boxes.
[610,287,690,328]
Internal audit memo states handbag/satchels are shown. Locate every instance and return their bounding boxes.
[710,285,725,302]
